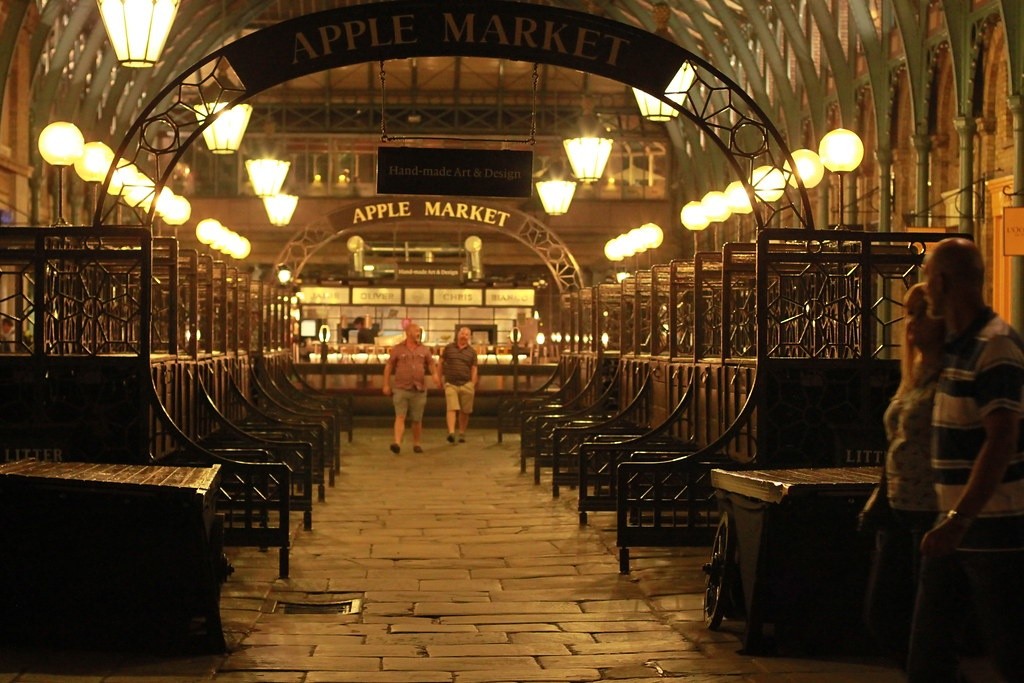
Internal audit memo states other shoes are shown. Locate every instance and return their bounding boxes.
[458,434,465,443]
[447,433,455,443]
[390,443,400,453]
[414,445,422,453]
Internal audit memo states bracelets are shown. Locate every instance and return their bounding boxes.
[947,510,969,530]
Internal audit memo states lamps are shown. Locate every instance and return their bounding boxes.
[38,0,865,262]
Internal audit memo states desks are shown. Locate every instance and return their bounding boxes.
[708,466,884,654]
[0,457,234,657]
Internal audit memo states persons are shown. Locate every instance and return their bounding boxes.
[902,238,1024,683]
[382,324,440,455]
[857,282,950,683]
[437,327,477,442]
[352,316,375,343]
